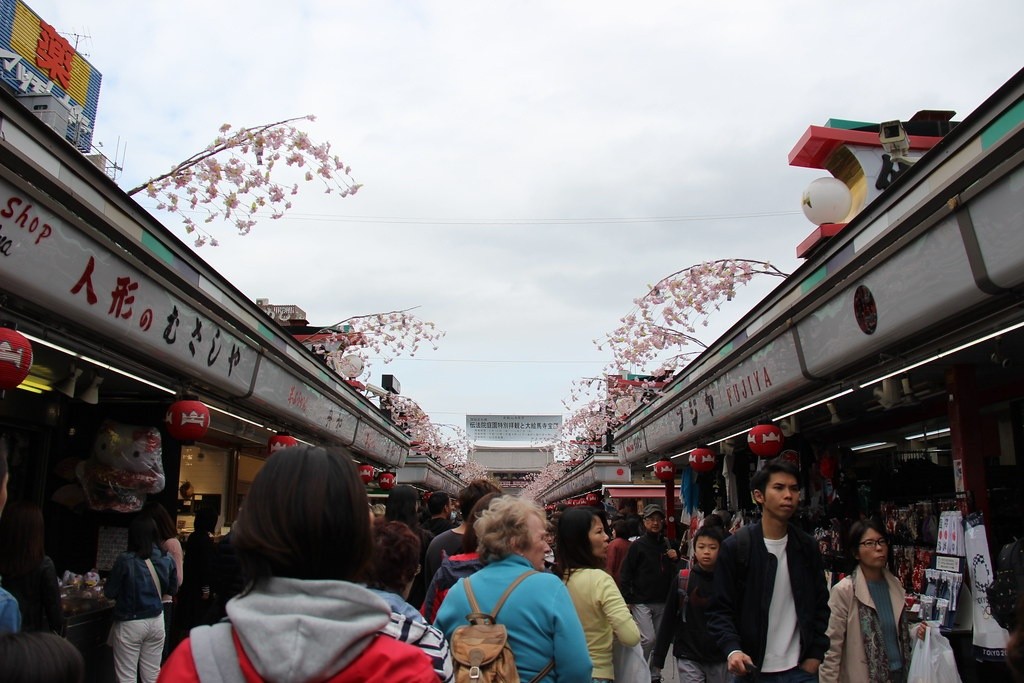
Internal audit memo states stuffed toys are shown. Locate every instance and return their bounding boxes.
[74,420,166,512]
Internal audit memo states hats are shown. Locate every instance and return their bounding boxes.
[643,504,665,518]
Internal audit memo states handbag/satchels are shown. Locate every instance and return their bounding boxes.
[906,620,963,683]
[106,621,114,647]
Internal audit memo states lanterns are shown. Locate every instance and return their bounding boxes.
[0,318,34,400]
[688,447,716,473]
[563,493,598,506]
[747,418,785,460]
[267,431,394,491]
[653,458,676,483]
[166,392,211,446]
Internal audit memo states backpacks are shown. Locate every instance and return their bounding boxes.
[448,570,555,683]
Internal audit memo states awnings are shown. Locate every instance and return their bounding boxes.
[607,488,666,499]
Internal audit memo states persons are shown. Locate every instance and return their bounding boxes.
[0,438,68,638]
[818,521,928,683]
[403,479,503,626]
[103,507,250,683]
[936,574,950,598]
[541,502,736,683]
[156,443,443,683]
[0,631,84,683]
[705,459,832,683]
[383,485,434,610]
[434,493,594,682]
[368,520,455,682]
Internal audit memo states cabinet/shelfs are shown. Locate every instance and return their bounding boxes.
[878,494,982,642]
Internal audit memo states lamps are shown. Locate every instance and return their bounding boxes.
[826,401,842,424]
[719,438,735,456]
[48,362,104,405]
[781,419,794,437]
[900,374,921,408]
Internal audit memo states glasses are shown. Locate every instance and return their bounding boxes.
[857,537,889,548]
[414,564,421,575]
